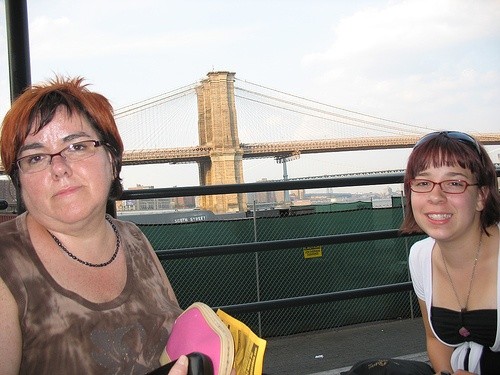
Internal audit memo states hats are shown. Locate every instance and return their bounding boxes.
[341,358,436,375]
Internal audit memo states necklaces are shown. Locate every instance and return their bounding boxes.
[438,231,482,317]
[46,215,120,267]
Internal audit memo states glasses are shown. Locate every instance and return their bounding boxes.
[407,178,478,194]
[13,139,120,173]
[413,131,487,151]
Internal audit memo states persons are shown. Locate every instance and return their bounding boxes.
[0,74,188,375]
[401,131,500,375]
